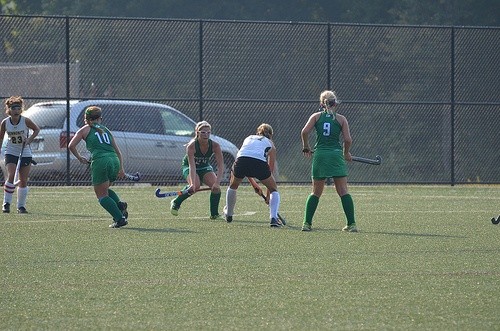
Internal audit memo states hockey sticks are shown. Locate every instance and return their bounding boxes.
[491,216,500,225]
[154,188,213,198]
[11,142,25,186]
[255,189,286,226]
[87,161,140,182]
[305,150,382,164]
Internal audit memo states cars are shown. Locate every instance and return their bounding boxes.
[0,98,240,188]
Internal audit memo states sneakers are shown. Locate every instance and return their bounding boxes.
[210,214,221,220]
[301,222,313,231]
[170,198,180,216]
[2,202,10,213]
[342,223,358,232]
[17,207,28,213]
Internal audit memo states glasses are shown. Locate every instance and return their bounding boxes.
[198,131,211,134]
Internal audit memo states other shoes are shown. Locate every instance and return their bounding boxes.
[269,217,282,227]
[118,201,128,219]
[109,220,128,228]
[223,206,232,222]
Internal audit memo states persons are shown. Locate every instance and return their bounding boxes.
[302,90,358,232]
[68,106,129,228]
[0,96,40,213]
[170,121,225,220]
[223,123,284,226]
